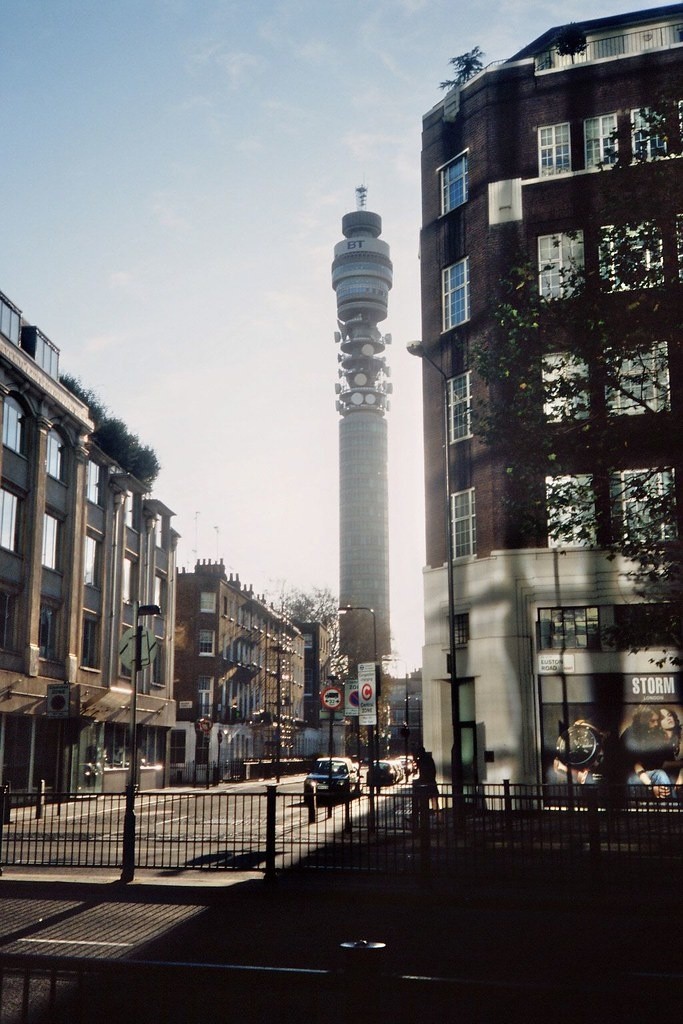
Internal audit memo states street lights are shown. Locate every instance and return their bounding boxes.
[120,604,163,881]
[336,605,382,801]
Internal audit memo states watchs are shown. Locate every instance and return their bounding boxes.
[551,721,604,799]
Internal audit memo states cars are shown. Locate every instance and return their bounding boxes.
[304,757,362,802]
[366,754,417,787]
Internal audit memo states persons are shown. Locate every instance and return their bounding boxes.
[621,706,683,806]
[416,747,440,817]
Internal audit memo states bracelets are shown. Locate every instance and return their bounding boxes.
[638,770,646,778]
